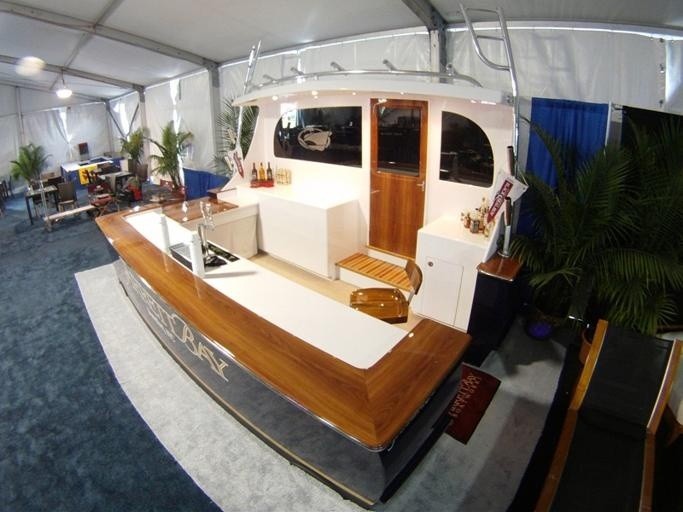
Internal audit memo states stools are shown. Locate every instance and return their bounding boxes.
[56,200,80,223]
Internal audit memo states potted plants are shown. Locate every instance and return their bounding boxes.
[496,113,683,367]
[116,119,195,204]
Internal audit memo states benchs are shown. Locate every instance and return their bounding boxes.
[41,204,96,230]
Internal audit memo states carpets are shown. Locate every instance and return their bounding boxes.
[444,364,502,445]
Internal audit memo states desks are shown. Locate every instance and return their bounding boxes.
[24,184,58,225]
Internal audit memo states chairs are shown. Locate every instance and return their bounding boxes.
[349,260,423,324]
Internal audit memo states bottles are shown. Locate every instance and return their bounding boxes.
[464,197,490,234]
[251,162,273,181]
[275,168,290,185]
